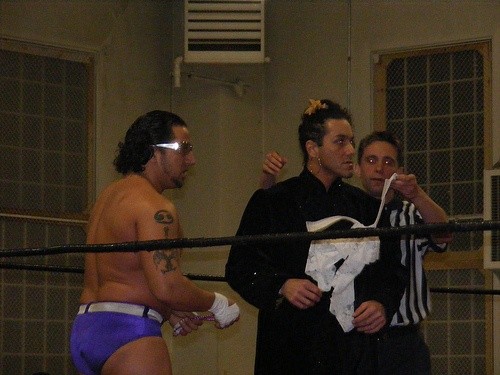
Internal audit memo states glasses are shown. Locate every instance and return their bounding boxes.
[148,140,191,156]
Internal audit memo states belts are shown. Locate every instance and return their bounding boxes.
[77,302,163,324]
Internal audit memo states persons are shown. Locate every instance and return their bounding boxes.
[259,131,455,375]
[225,99,407,375]
[70,110,241,375]
